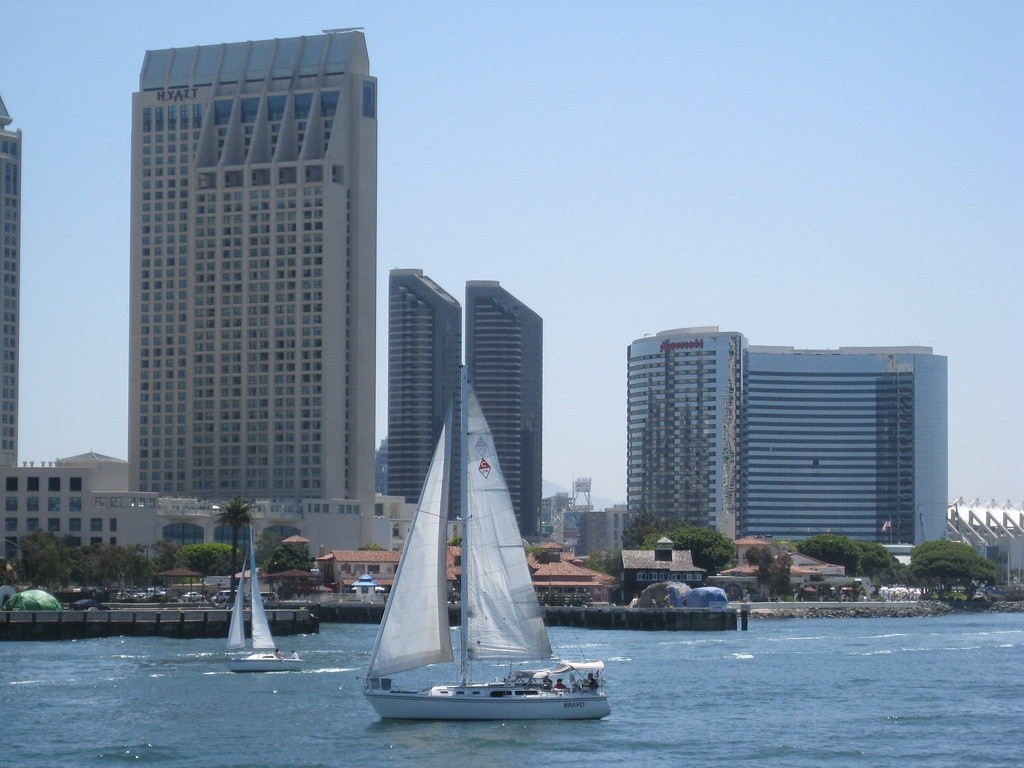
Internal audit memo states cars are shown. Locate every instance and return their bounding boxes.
[71,598,110,610]
[181,591,207,602]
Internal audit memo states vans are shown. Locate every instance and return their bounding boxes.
[218,590,248,603]
[259,592,279,601]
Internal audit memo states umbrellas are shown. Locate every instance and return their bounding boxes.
[804,587,816,593]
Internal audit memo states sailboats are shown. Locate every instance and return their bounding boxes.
[222,525,307,673]
[362,362,613,722]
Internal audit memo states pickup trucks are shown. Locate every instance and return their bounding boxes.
[137,587,166,597]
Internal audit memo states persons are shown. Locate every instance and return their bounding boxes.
[553,679,566,691]
[586,674,599,690]
[632,594,687,607]
[274,648,298,659]
[544,678,553,691]
